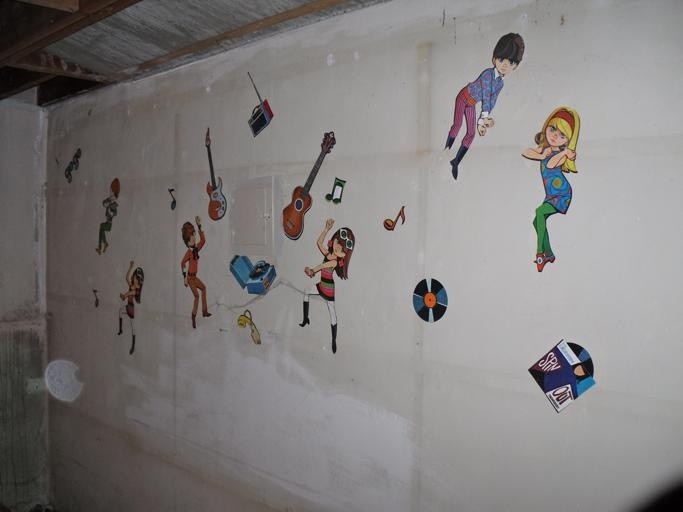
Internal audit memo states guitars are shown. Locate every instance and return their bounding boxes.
[282,131,336,239]
[205,127,227,220]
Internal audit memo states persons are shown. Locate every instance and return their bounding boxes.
[298,219,356,354]
[180,216,212,330]
[443,34,522,180]
[116,260,144,355]
[522,106,581,272]
[94,175,120,255]
